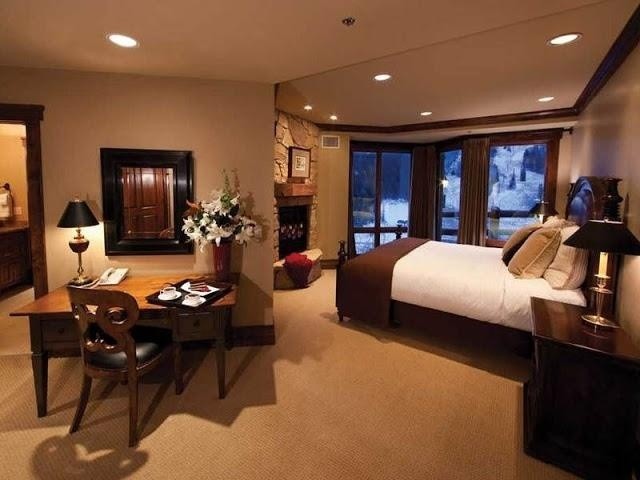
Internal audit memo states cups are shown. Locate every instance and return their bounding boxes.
[159,286,176,296]
[185,293,201,301]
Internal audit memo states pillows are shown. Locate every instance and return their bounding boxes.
[508,227,561,279]
[502,223,541,265]
[543,227,589,290]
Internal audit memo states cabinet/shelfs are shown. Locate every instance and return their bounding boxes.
[0,225,31,296]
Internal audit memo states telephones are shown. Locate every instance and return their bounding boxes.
[97,266,130,285]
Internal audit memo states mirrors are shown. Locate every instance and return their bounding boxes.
[99,147,196,257]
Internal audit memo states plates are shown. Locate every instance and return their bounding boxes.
[157,292,182,301]
[180,282,220,296]
[182,296,207,307]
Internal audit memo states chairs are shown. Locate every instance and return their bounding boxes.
[66,285,183,447]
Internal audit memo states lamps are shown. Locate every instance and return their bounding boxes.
[562,216,640,331]
[56,194,101,286]
[530,201,560,223]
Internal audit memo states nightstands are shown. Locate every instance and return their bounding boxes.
[523,296,640,480]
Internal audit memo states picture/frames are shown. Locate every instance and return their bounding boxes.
[287,146,312,178]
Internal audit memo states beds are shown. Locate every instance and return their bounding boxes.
[334,174,623,356]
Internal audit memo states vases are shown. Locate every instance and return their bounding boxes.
[213,242,232,282]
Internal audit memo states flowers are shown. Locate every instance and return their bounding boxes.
[182,169,264,252]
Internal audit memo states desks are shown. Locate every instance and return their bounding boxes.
[9,276,242,419]
